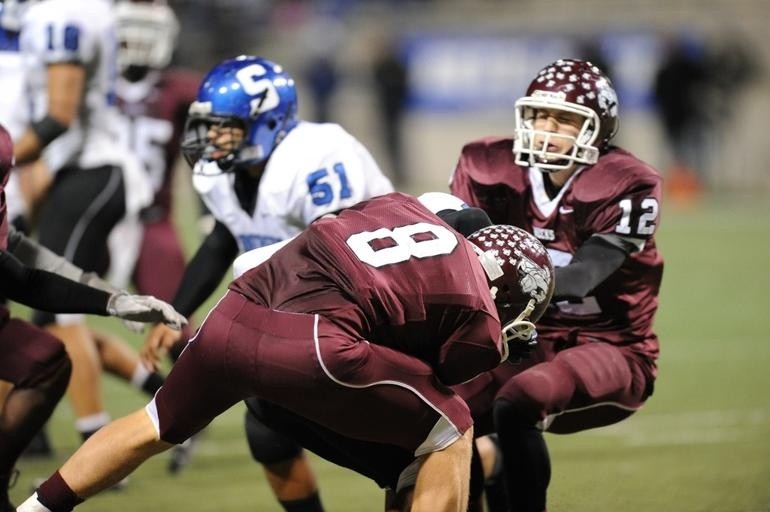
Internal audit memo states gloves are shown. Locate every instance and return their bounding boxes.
[108,293,190,332]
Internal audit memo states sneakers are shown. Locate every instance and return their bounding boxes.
[168,429,198,472]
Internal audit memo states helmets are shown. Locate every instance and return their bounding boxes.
[468,223,555,364]
[181,55,297,178]
[513,57,618,169]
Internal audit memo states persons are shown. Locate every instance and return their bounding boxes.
[0,1,759,512]
